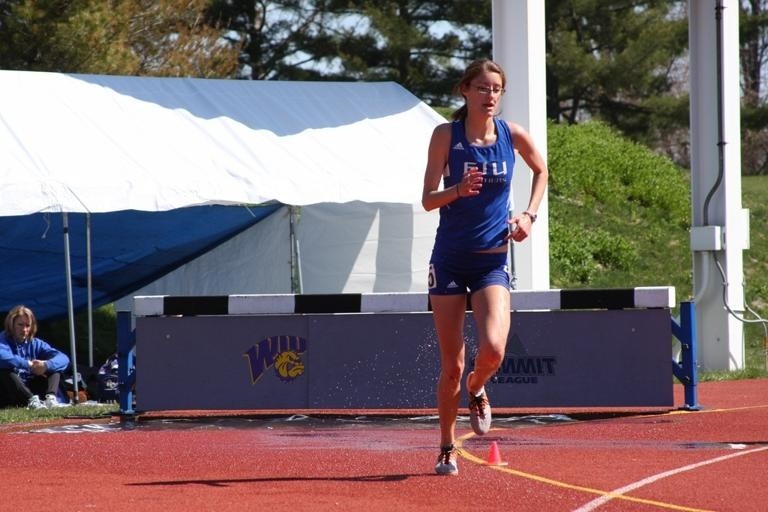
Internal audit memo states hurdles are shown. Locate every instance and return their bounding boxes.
[113,287,703,416]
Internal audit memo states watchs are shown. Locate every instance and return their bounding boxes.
[522,211,538,223]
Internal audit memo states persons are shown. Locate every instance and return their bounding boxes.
[0,305,70,411]
[421,56,550,475]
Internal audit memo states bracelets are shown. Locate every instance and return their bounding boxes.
[456,183,462,199]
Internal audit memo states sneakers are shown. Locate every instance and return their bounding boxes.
[466,371,491,434]
[435,440,458,477]
[27,393,63,411]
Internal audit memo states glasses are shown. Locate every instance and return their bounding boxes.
[469,82,506,95]
